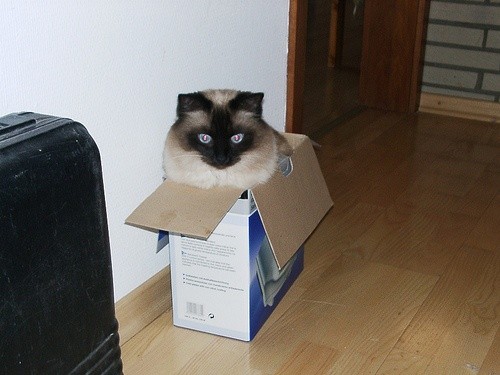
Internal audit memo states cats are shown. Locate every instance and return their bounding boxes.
[162,89,293,188]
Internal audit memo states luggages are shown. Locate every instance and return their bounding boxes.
[1,112,123,375]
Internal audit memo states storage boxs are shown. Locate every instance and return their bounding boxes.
[126,130,335,342]
